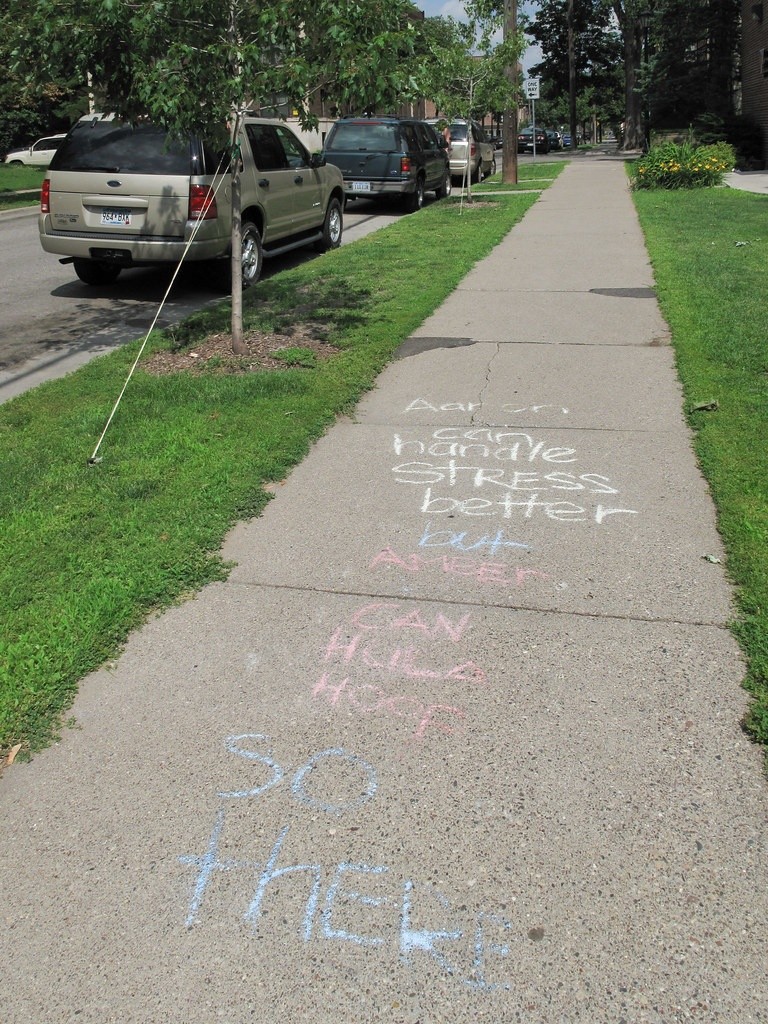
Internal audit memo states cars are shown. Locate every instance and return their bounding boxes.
[496,137,503,150]
[517,127,591,153]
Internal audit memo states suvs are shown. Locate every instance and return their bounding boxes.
[37,110,346,292]
[4,133,68,167]
[322,117,453,214]
[419,119,497,187]
